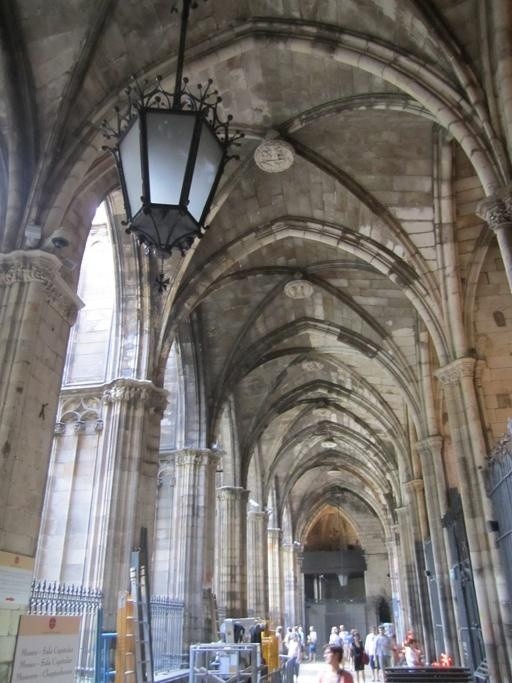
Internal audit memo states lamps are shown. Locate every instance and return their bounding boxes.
[98,0,248,296]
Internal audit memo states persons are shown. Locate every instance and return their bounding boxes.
[234,624,424,683]
